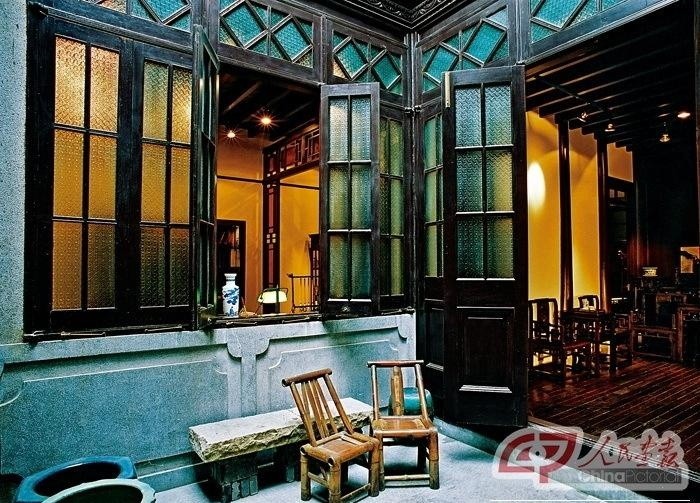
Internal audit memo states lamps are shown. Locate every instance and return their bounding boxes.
[253,287,289,315]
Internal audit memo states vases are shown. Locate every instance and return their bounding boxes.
[222,272,240,317]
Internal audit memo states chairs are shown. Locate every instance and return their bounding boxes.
[282,369,379,503]
[366,359,440,489]
[527,286,700,389]
